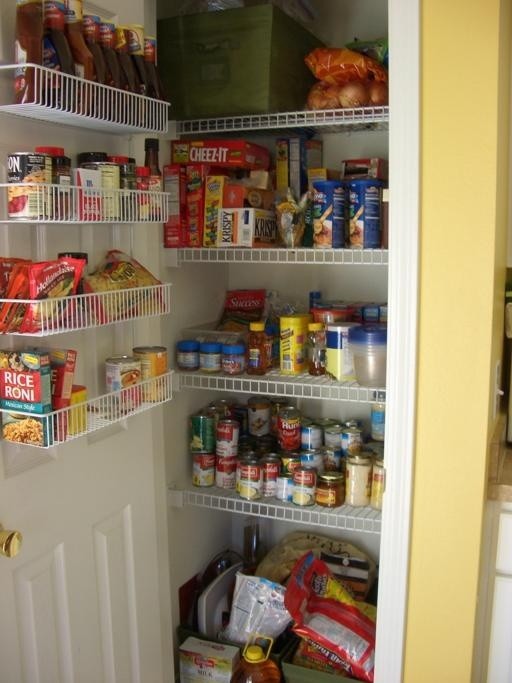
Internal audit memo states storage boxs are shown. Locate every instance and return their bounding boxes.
[179,622,296,670]
[156,2,327,121]
[280,636,373,681]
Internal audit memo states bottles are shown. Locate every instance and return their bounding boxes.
[381,189,389,249]
[305,321,326,375]
[126,23,151,126]
[346,178,382,250]
[34,145,73,219]
[188,390,386,511]
[199,341,222,373]
[313,179,349,248]
[104,354,143,412]
[246,320,267,375]
[279,312,315,376]
[349,323,388,390]
[144,137,163,220]
[115,27,142,125]
[175,339,200,371]
[5,151,53,221]
[77,151,150,220]
[64,0,95,115]
[99,21,122,121]
[221,343,245,375]
[83,13,108,115]
[325,321,362,382]
[133,345,168,401]
[143,36,163,127]
[13,1,43,104]
[230,644,282,683]
[43,0,78,111]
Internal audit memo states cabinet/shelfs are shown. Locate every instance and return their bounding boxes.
[3,44,413,680]
